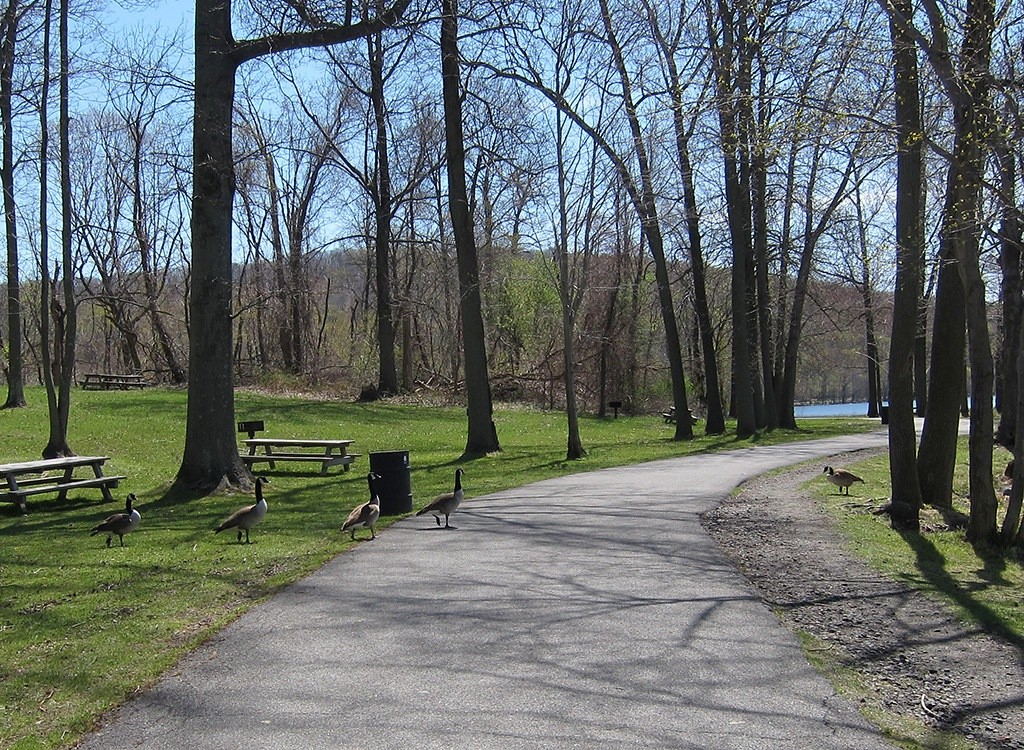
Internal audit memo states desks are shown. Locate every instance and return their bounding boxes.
[241,439,356,476]
[0,455,114,515]
[82,373,144,390]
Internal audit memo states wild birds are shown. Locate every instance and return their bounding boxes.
[90,492,141,549]
[416,468,466,530]
[340,472,382,540]
[212,476,271,544]
[823,466,865,496]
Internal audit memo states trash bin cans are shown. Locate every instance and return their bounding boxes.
[369,450,413,518]
[881,407,889,424]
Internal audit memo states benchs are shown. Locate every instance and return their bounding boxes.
[77,380,146,386]
[0,475,127,496]
[240,451,362,463]
